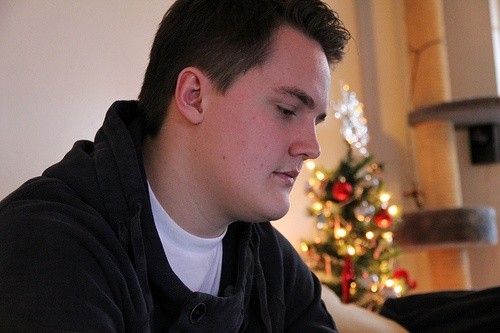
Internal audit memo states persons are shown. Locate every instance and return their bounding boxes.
[0,0,354,333]
